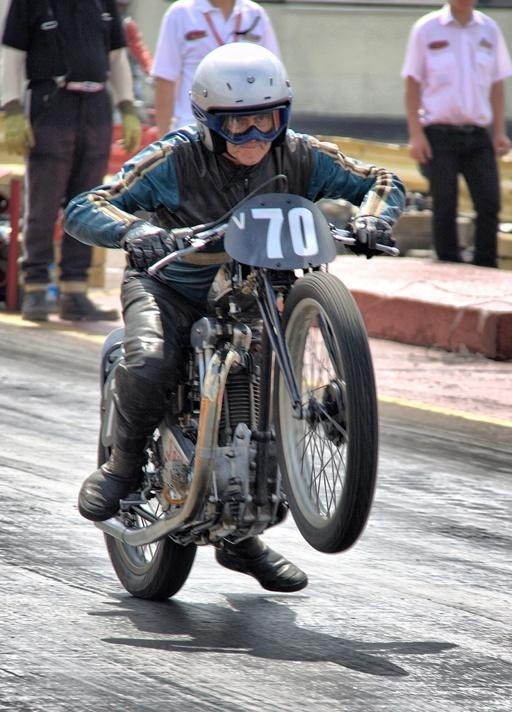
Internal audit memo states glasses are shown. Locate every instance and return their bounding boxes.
[191,101,290,144]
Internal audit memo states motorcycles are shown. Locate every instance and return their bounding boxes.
[85,170,401,604]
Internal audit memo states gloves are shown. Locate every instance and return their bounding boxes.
[344,215,396,259]
[121,219,177,273]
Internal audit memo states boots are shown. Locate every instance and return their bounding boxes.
[78,363,166,522]
[216,536,308,592]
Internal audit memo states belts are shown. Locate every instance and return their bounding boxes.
[66,81,105,93]
[430,124,488,134]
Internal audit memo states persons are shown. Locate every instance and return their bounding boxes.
[403,0,512,267]
[113,0,153,99]
[62,42,407,592]
[0,0,144,319]
[150,0,278,140]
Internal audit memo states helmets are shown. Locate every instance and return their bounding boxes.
[188,41,293,154]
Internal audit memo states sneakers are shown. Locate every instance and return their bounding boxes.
[21,291,120,321]
